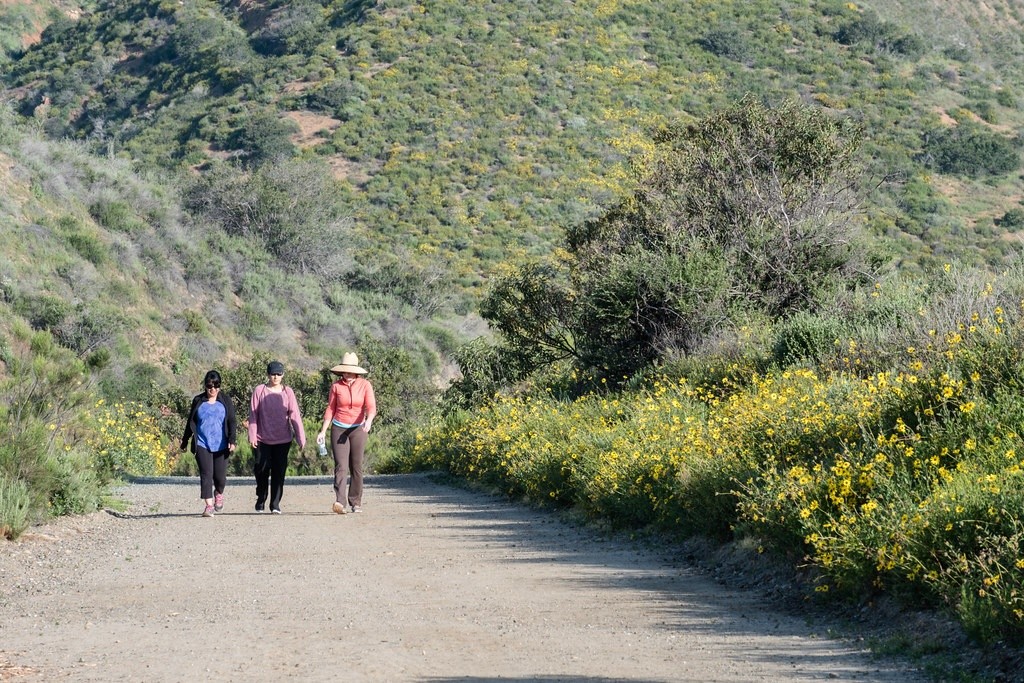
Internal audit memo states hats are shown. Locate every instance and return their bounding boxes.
[330,352,368,376]
[267,362,284,374]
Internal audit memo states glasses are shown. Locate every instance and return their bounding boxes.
[205,383,220,389]
[272,374,282,376]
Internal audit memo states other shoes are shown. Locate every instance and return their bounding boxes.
[256,499,264,512]
[332,502,347,514]
[271,507,281,514]
[352,505,362,513]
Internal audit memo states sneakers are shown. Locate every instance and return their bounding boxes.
[202,505,215,518]
[214,489,224,512]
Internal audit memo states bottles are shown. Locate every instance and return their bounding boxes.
[318,435,327,456]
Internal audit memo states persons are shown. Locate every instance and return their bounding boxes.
[179,371,236,517]
[316,352,376,513]
[248,361,305,514]
